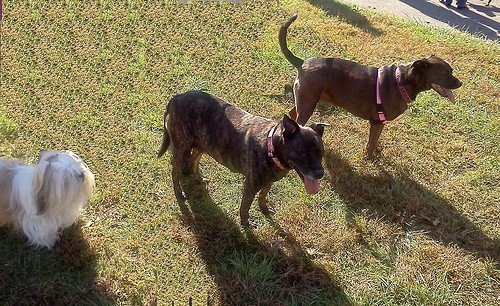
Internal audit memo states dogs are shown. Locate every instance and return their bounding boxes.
[157,90,330,225]
[0,149,95,250]
[278,14,463,153]
